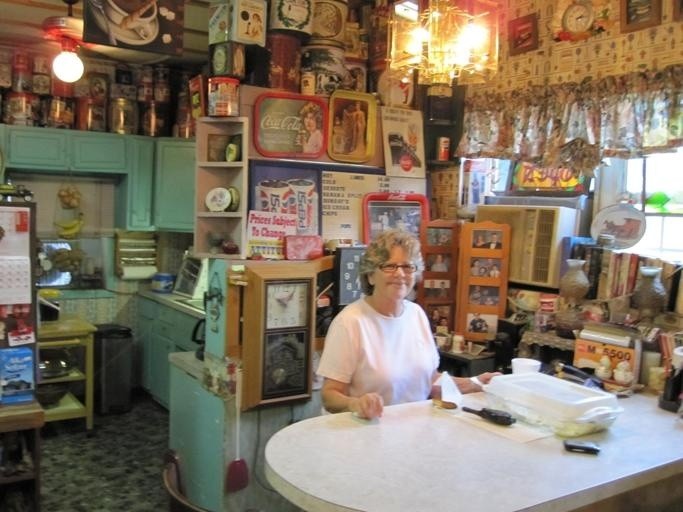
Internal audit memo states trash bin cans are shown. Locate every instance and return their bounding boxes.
[93,323,134,413]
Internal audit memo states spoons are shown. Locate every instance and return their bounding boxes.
[92,0,117,46]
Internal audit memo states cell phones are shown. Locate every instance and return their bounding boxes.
[565,438,600,452]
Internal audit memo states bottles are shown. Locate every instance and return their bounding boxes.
[141,99,166,137]
[359,27,369,61]
[453,332,466,353]
[300,70,316,94]
[436,136,450,163]
[11,52,35,94]
[223,239,238,254]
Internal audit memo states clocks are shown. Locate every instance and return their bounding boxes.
[241,267,316,413]
[562,0,595,34]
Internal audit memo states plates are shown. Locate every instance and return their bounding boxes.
[91,0,160,47]
[590,201,646,251]
[40,357,78,377]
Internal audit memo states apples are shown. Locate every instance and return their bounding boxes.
[58,184,81,208]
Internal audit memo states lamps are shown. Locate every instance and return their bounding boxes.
[41,0,84,83]
[386,0,500,97]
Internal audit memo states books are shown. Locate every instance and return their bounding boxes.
[560,235,683,315]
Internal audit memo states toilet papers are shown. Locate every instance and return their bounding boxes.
[119,265,158,280]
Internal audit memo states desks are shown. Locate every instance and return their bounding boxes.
[518,329,576,365]
[264,371,683,512]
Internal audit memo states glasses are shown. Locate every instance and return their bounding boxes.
[376,262,418,273]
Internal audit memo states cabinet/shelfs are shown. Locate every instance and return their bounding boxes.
[134,291,205,412]
[37,314,96,432]
[155,118,258,313]
[0,123,154,297]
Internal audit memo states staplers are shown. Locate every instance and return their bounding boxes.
[550,360,605,389]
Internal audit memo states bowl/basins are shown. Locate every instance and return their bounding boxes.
[555,312,586,338]
[38,384,72,409]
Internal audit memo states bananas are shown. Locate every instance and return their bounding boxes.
[54,211,84,239]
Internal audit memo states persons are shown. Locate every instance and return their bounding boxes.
[425,281,450,300]
[471,258,501,278]
[300,103,324,153]
[469,286,494,305]
[378,209,418,233]
[346,103,367,156]
[315,230,502,420]
[393,153,420,175]
[473,233,503,250]
[429,307,448,333]
[430,254,450,273]
[468,313,488,333]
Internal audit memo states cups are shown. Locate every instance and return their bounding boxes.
[511,358,542,372]
[641,351,662,388]
[434,334,452,352]
[106,0,158,30]
[208,230,224,255]
[258,179,316,233]
